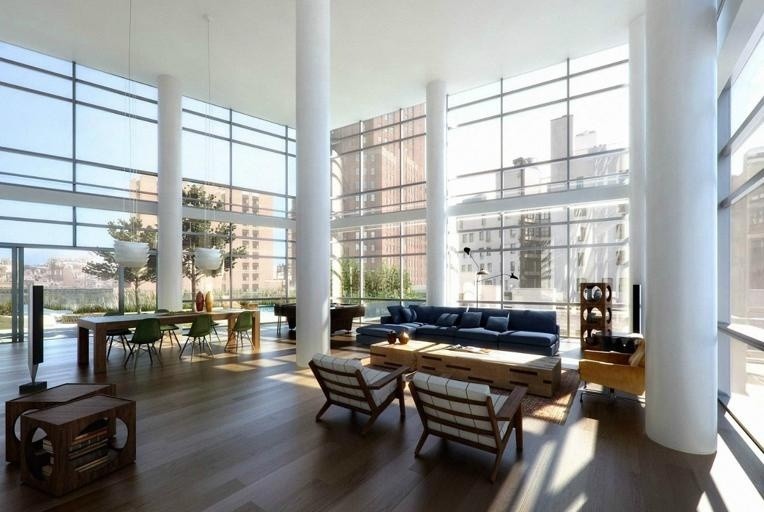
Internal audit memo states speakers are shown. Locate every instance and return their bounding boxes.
[633,284,641,333]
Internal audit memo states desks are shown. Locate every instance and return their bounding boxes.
[74,307,260,375]
[273,301,367,339]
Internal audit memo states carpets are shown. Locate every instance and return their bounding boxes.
[356,356,583,427]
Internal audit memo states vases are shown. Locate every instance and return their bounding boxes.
[195,290,204,311]
[204,290,214,312]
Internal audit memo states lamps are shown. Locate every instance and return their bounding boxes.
[192,12,225,272]
[463,245,519,309]
[112,0,149,275]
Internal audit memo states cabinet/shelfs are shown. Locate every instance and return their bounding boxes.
[578,282,613,350]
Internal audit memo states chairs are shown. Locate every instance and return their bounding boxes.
[403,366,530,483]
[581,331,645,355]
[195,312,220,345]
[307,352,411,444]
[576,337,647,414]
[151,308,182,354]
[221,310,256,354]
[176,315,214,364]
[122,317,164,375]
[102,310,134,360]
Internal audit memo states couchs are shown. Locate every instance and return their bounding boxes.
[354,303,561,356]
